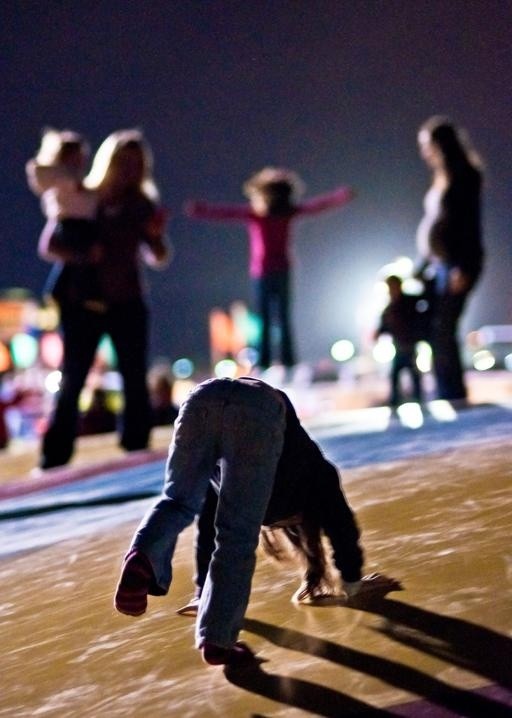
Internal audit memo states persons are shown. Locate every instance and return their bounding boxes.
[82,385,115,437]
[23,121,98,301]
[148,374,182,429]
[179,161,362,389]
[410,109,495,405]
[366,270,429,412]
[107,373,406,671]
[33,125,175,475]
